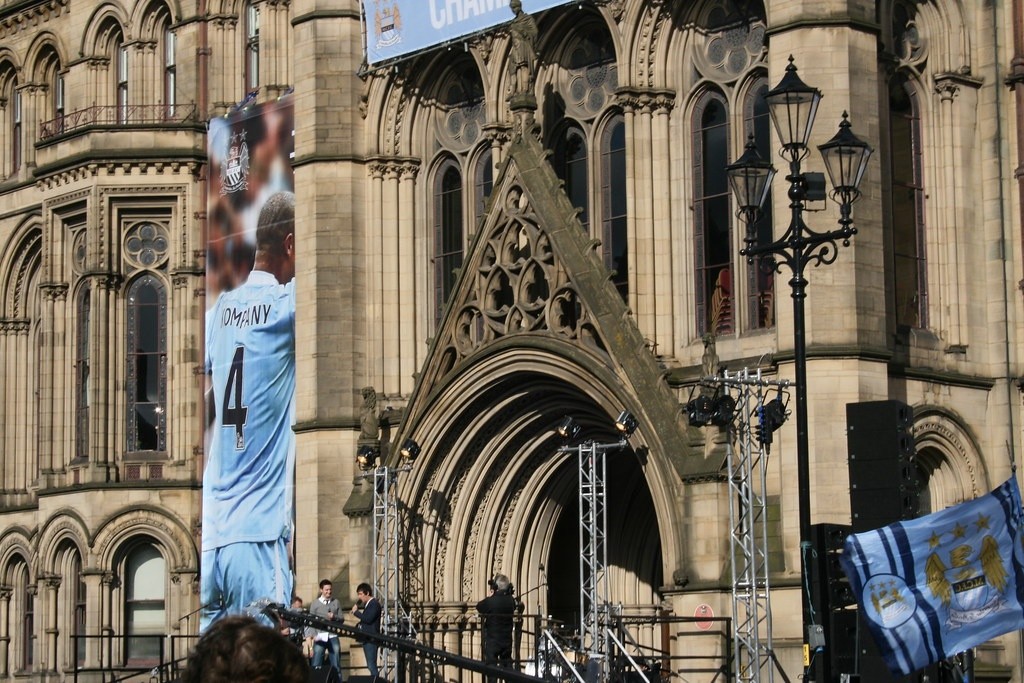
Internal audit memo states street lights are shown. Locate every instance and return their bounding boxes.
[719,54,876,683]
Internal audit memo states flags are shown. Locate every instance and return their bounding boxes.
[841,477,1024,677]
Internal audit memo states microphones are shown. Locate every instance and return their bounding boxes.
[350,598,363,615]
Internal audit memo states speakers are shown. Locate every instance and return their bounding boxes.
[800,400,946,683]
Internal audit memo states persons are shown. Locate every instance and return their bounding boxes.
[200,96,294,632]
[352,582,382,677]
[181,616,309,683]
[309,579,345,680]
[476,574,516,683]
[291,598,314,658]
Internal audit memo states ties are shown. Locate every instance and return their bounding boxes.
[325,600,329,605]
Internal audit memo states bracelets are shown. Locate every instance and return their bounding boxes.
[309,647,313,650]
